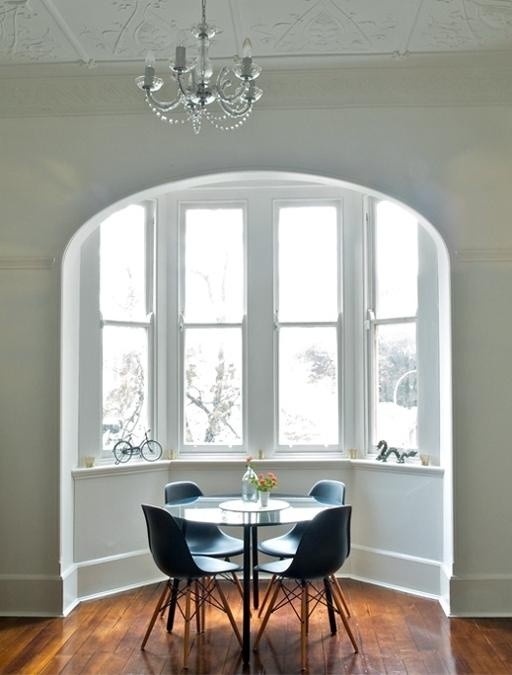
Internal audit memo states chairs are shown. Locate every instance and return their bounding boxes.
[160,480,253,617]
[139,503,247,668]
[254,504,360,673]
[258,479,355,618]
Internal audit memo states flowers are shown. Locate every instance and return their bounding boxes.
[250,473,280,492]
[244,455,255,468]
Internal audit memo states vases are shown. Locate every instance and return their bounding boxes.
[259,490,270,508]
